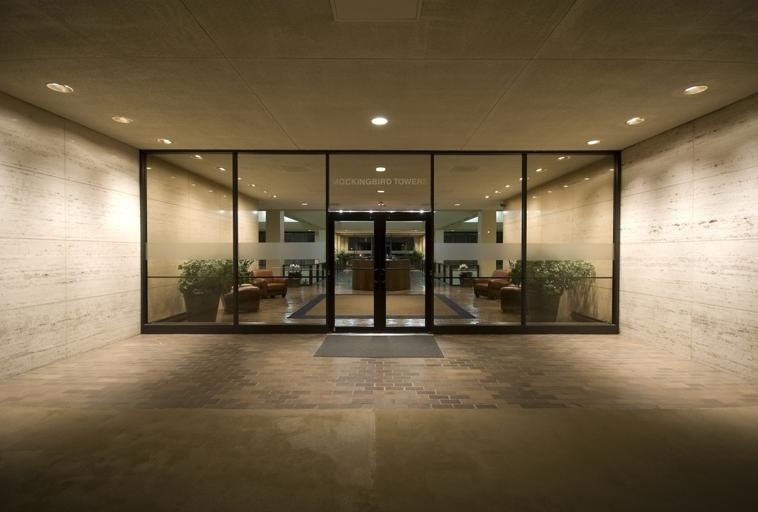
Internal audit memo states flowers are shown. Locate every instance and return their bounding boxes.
[290,263,302,277]
[458,264,472,277]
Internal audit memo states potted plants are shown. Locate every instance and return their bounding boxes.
[179,259,221,323]
[512,261,594,322]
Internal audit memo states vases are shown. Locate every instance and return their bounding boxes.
[460,278,472,287]
[289,277,300,287]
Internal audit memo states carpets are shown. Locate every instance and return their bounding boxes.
[313,333,444,358]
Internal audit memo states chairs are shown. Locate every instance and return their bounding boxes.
[221,269,288,314]
[472,269,524,313]
